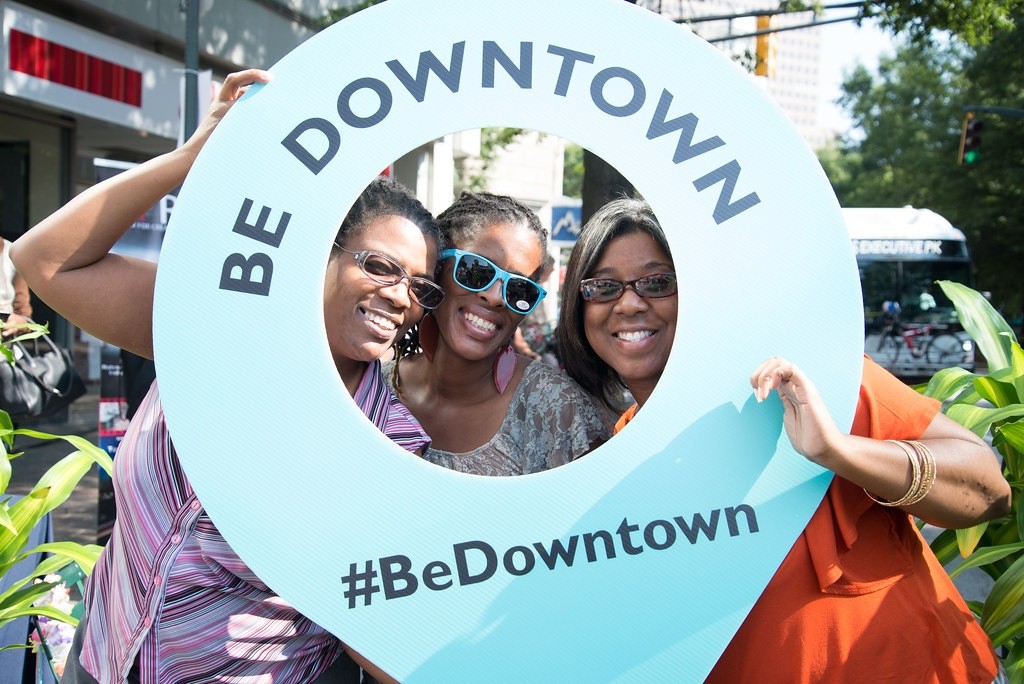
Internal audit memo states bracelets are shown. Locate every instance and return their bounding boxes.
[863,440,938,508]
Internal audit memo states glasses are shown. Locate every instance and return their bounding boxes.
[335,242,446,311]
[579,273,678,303]
[437,248,547,315]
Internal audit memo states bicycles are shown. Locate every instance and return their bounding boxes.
[862,303,967,372]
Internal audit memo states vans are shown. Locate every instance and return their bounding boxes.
[838,203,993,377]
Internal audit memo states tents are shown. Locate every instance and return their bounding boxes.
[839,205,968,293]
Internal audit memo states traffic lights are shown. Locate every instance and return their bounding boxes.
[954,113,986,170]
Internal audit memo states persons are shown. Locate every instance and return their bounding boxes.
[883,293,901,340]
[360,190,611,684]
[0,70,444,684]
[558,195,1011,684]
[919,286,936,324]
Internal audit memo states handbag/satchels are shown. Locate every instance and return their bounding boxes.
[0,316,86,424]
